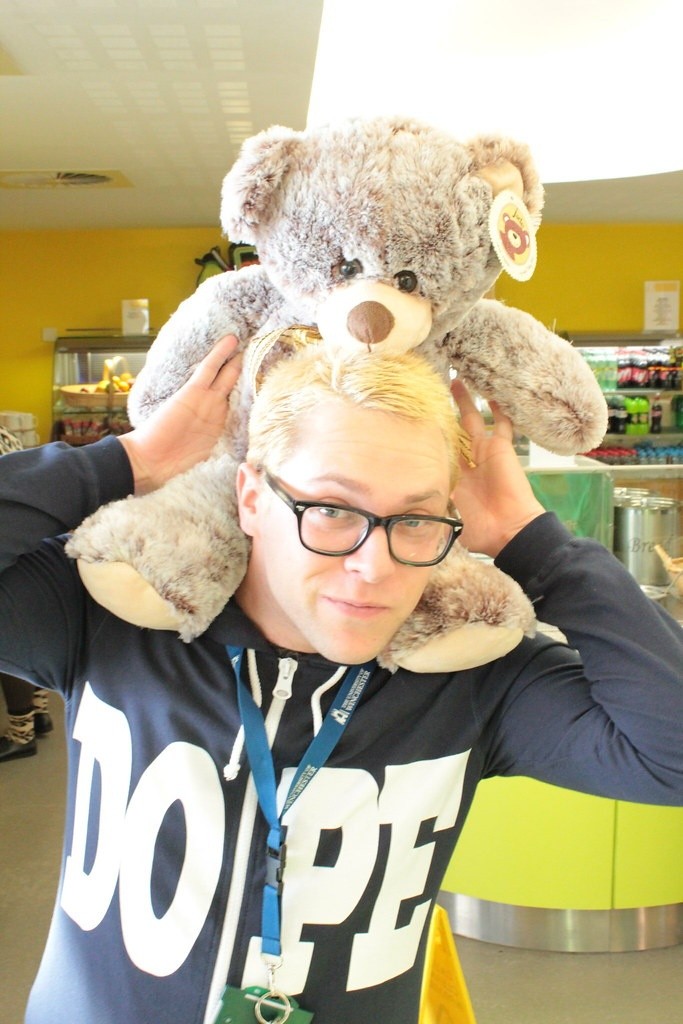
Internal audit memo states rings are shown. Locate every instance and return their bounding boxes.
[468,462,476,468]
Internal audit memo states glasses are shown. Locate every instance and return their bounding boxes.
[257,468,463,568]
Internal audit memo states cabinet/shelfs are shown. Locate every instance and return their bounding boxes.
[601,389,683,448]
[52,334,156,446]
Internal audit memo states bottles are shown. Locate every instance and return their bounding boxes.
[579,442,683,465]
[574,342,683,388]
[603,392,683,436]
[121,298,149,336]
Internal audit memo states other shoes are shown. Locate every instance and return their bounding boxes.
[0,735,37,762]
[32,712,54,735]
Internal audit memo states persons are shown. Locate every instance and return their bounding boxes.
[0,426,53,760]
[0,335,682,1024]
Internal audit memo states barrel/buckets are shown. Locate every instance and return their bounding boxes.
[613,487,682,586]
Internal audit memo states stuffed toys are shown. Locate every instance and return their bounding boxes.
[64,121,610,675]
